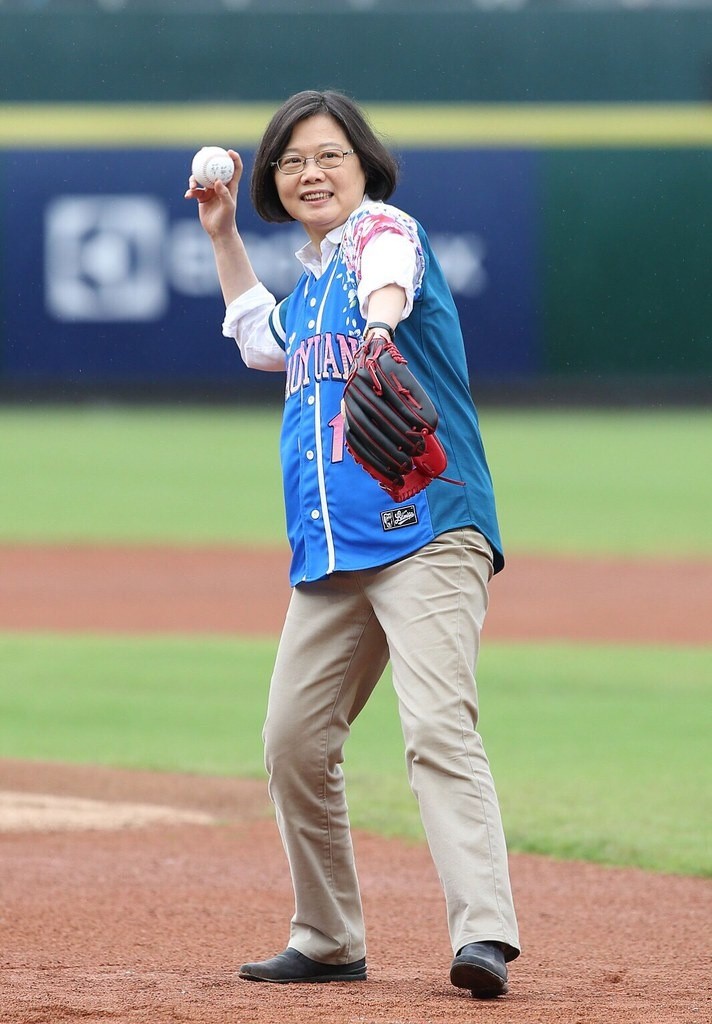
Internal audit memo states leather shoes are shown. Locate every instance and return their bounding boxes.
[449,943,509,999]
[238,946,368,983]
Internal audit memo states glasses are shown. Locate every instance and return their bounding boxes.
[271,148,357,175]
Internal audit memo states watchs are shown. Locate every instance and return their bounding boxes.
[362,322,396,341]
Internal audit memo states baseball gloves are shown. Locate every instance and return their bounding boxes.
[339,328,470,501]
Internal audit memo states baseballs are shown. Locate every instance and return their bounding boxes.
[192,144,239,189]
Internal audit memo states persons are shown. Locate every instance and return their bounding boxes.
[184,90,520,999]
